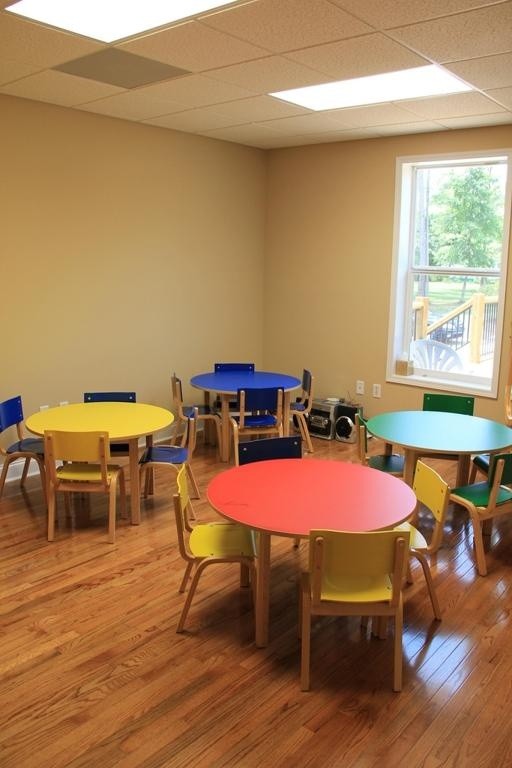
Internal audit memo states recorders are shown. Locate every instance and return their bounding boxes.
[293,397,364,444]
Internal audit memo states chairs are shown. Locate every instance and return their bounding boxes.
[360,458,450,637]
[84,392,136,495]
[172,461,257,633]
[0,395,69,500]
[44,430,127,544]
[171,362,315,464]
[299,528,411,691]
[422,393,474,417]
[411,340,462,372]
[138,407,201,520]
[468,454,490,485]
[447,452,512,576]
[355,414,405,477]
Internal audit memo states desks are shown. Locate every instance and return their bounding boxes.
[206,459,417,647]
[25,402,175,525]
[366,411,512,529]
